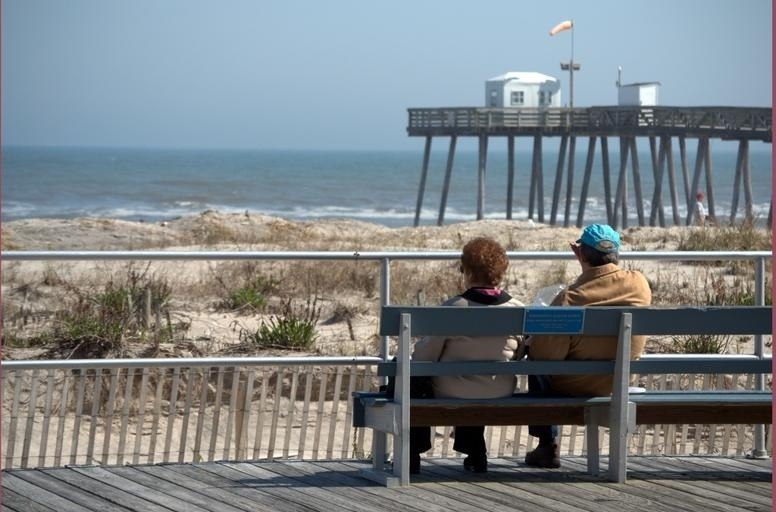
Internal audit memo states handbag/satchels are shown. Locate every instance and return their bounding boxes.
[386,356,435,400]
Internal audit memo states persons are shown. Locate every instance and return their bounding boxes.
[410,234,527,476]
[524,223,654,469]
[692,191,714,228]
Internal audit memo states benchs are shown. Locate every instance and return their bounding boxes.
[350,306,773,487]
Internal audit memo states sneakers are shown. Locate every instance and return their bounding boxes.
[409,452,420,474]
[462,452,489,474]
[524,438,562,469]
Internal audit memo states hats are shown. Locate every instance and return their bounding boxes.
[575,222,621,254]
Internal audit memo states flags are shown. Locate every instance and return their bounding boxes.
[548,21,572,38]
